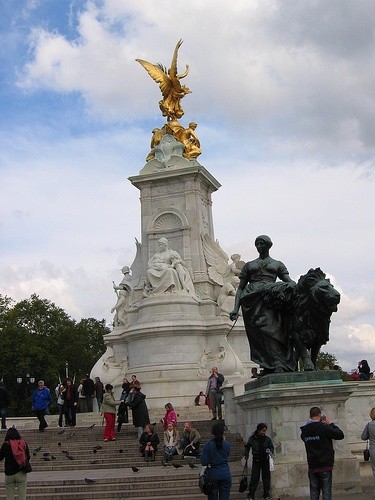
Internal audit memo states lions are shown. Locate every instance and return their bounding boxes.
[254,265,342,372]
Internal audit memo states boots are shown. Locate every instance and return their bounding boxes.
[144,450,150,461]
[150,450,155,461]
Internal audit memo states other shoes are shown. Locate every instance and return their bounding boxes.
[104,438,109,442]
[111,437,117,441]
[218,416,223,421]
[212,416,217,420]
[246,495,255,500]
[263,495,272,500]
[57,395,64,406]
[164,451,173,460]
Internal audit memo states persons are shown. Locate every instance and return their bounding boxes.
[146,126,166,161]
[222,253,245,295]
[195,367,224,420]
[251,368,259,378]
[244,423,274,500]
[111,266,131,327]
[324,360,370,380]
[300,407,345,500]
[102,375,150,442]
[156,65,192,122]
[201,422,232,500]
[55,378,79,427]
[0,376,10,429]
[146,237,185,294]
[32,380,52,432]
[0,427,33,500]
[76,373,105,413]
[229,235,314,376]
[139,403,200,466]
[177,122,202,160]
[361,408,375,478]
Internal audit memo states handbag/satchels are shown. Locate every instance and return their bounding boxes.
[364,450,370,461]
[239,477,248,493]
[184,444,197,459]
[198,475,213,495]
[21,443,33,473]
[268,454,276,472]
[117,414,129,423]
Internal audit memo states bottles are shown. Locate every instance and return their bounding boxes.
[266,448,270,459]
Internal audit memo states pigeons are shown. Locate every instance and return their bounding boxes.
[87,424,96,431]
[188,462,199,470]
[32,446,42,456]
[171,462,184,470]
[83,477,97,485]
[152,422,157,426]
[119,448,123,454]
[65,431,76,441]
[40,452,57,462]
[57,429,65,436]
[57,441,61,447]
[92,445,102,453]
[130,465,141,474]
[61,449,73,460]
[89,460,99,464]
